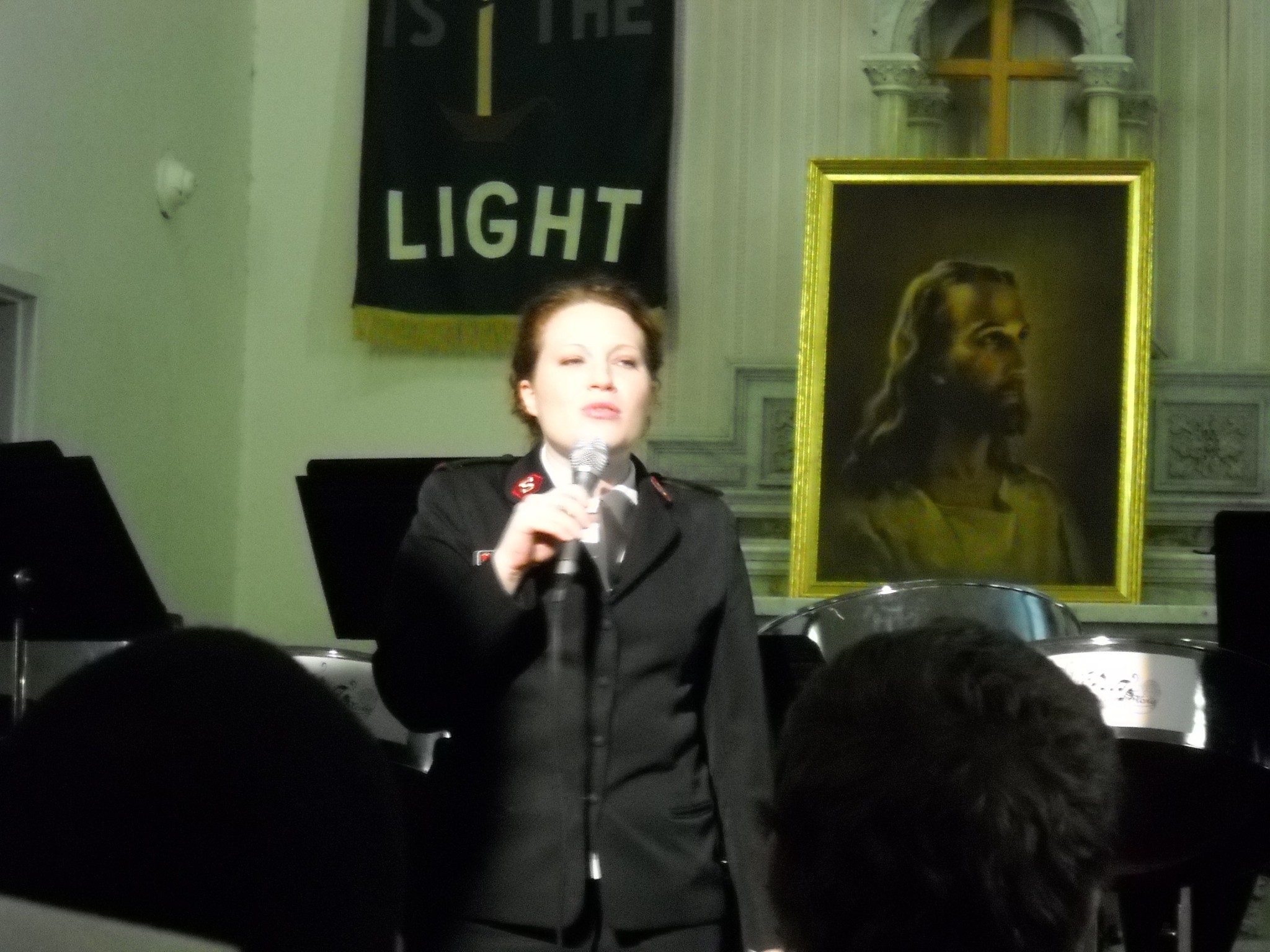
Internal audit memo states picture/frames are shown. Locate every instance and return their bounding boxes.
[788,157,1157,603]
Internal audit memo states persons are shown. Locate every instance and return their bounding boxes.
[371,275,779,952]
[764,620,1120,952]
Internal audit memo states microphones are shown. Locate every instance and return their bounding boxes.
[553,437,609,603]
